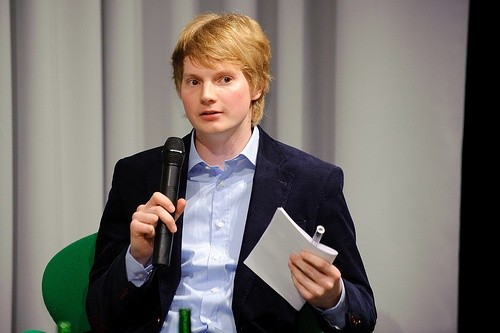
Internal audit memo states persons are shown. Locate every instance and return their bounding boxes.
[86,11,378,333]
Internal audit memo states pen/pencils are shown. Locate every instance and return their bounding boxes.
[311,224,325,243]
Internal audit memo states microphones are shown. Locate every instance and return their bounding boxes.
[156,137,185,266]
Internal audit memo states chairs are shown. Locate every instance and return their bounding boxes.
[41,232,98,332]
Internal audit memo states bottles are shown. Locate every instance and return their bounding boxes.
[57,320,71,332]
[178,307,192,333]
